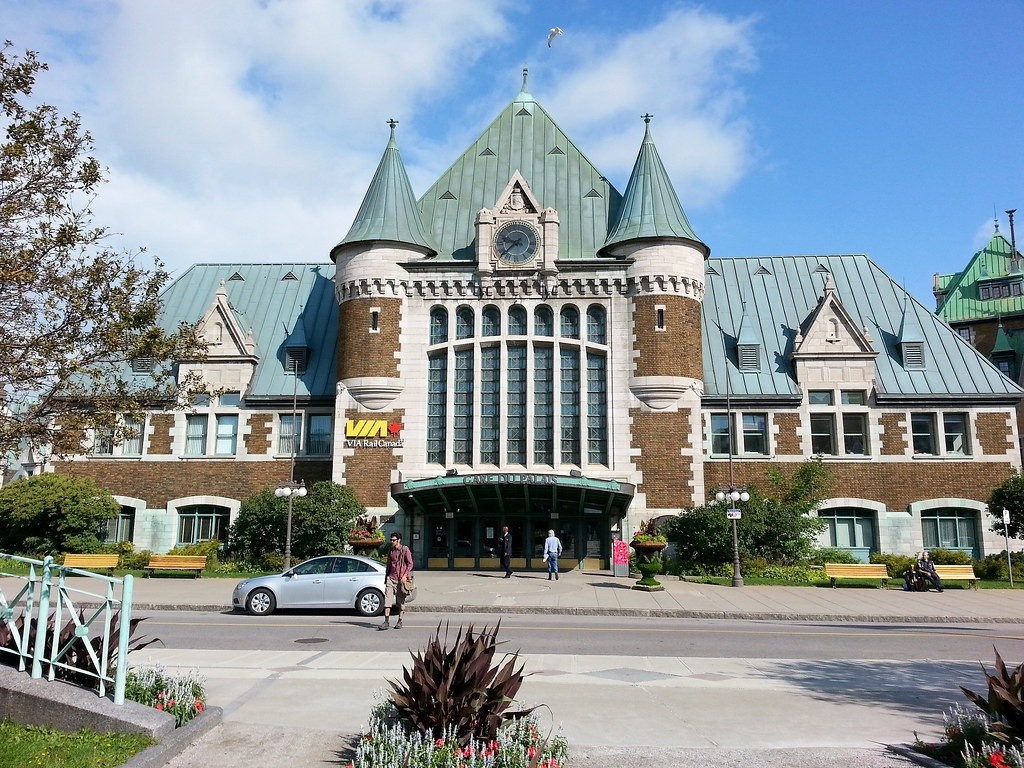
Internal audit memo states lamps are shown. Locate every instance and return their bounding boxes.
[570,470,582,477]
[446,468,458,476]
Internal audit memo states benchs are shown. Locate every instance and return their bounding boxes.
[62,554,119,576]
[144,554,208,579]
[825,564,892,589]
[911,565,981,591]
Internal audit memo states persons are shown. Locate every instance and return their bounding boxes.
[543,529,562,580]
[379,534,412,631]
[497,526,514,578]
[917,552,945,593]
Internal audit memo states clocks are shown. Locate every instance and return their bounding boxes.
[493,219,542,267]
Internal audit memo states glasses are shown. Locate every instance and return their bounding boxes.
[390,538,398,542]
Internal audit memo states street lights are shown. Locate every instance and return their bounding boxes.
[275,479,307,575]
[714,486,750,590]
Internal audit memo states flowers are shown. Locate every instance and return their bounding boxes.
[350,524,385,540]
[633,517,667,543]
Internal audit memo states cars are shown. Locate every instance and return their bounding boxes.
[230,555,417,616]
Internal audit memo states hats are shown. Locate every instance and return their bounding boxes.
[548,529,554,537]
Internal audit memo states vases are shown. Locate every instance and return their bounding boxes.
[348,538,384,559]
[631,539,667,585]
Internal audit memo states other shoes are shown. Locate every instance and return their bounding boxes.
[505,574,510,578]
[510,570,513,574]
[555,573,558,580]
[548,578,551,580]
[394,621,402,629]
[378,621,389,630]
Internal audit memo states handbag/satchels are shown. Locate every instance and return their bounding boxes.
[398,578,414,595]
